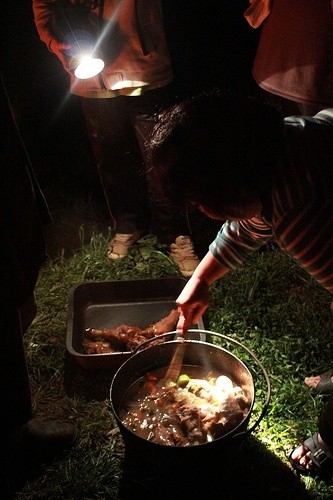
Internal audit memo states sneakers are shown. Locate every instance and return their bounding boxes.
[106,230,141,260]
[168,236,200,278]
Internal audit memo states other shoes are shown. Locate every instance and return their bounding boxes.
[6,420,79,455]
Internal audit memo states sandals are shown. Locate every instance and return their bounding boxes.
[289,433,333,473]
[304,369,333,401]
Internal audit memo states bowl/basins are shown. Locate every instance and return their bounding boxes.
[65,277,208,374]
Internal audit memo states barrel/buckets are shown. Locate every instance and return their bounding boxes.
[110,329,272,458]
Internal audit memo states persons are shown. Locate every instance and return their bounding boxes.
[0,72,79,497]
[32,0,200,277]
[145,92,333,472]
[245,0,333,116]
